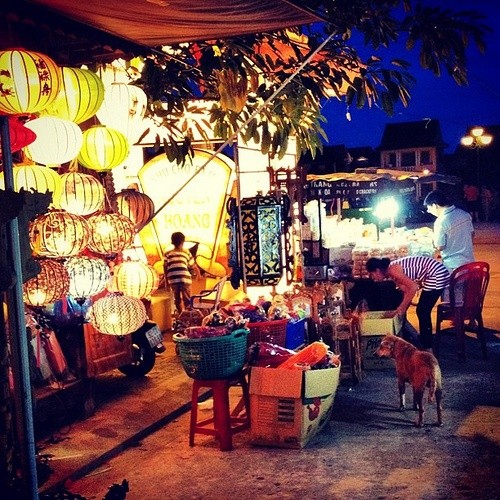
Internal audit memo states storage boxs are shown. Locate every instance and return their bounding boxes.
[285,317,306,349]
[249,356,341,450]
[361,332,401,369]
[303,249,328,279]
[359,310,404,336]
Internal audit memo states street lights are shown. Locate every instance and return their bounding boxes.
[460,125,493,221]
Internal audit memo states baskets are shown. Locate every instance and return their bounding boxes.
[172,327,249,380]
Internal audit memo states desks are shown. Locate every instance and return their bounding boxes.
[151,297,172,333]
[307,278,404,312]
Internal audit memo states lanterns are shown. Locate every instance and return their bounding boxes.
[0,48,156,336]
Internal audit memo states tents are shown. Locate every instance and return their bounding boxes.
[305,167,460,264]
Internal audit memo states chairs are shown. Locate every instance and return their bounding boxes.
[189,276,227,315]
[436,261,490,363]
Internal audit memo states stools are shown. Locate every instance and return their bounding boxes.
[320,317,359,385]
[189,372,251,451]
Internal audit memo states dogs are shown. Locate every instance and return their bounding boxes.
[373,332,445,428]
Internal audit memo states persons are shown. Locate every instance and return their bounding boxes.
[163,233,202,316]
[365,256,452,354]
[423,192,476,333]
